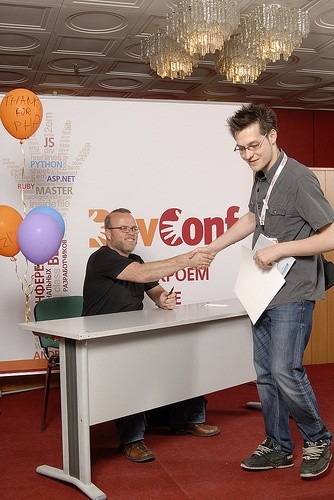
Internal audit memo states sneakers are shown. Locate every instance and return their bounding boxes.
[299,440,332,477]
[240,439,294,469]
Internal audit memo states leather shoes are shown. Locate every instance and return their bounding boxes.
[173,423,220,436]
[117,440,155,461]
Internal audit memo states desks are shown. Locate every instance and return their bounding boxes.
[18,298,263,500]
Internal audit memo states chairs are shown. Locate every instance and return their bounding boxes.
[34,296,83,431]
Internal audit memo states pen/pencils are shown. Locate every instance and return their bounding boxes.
[166,286,174,300]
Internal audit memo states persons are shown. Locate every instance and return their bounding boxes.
[81,208,221,461]
[187,103,334,478]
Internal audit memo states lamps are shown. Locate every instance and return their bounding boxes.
[139,0,309,84]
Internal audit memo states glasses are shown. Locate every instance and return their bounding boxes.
[233,132,269,154]
[107,226,140,234]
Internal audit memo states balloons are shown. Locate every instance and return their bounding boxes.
[0,205,65,267]
[0,89,43,144]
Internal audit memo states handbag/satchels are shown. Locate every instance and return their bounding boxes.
[324,262,334,291]
